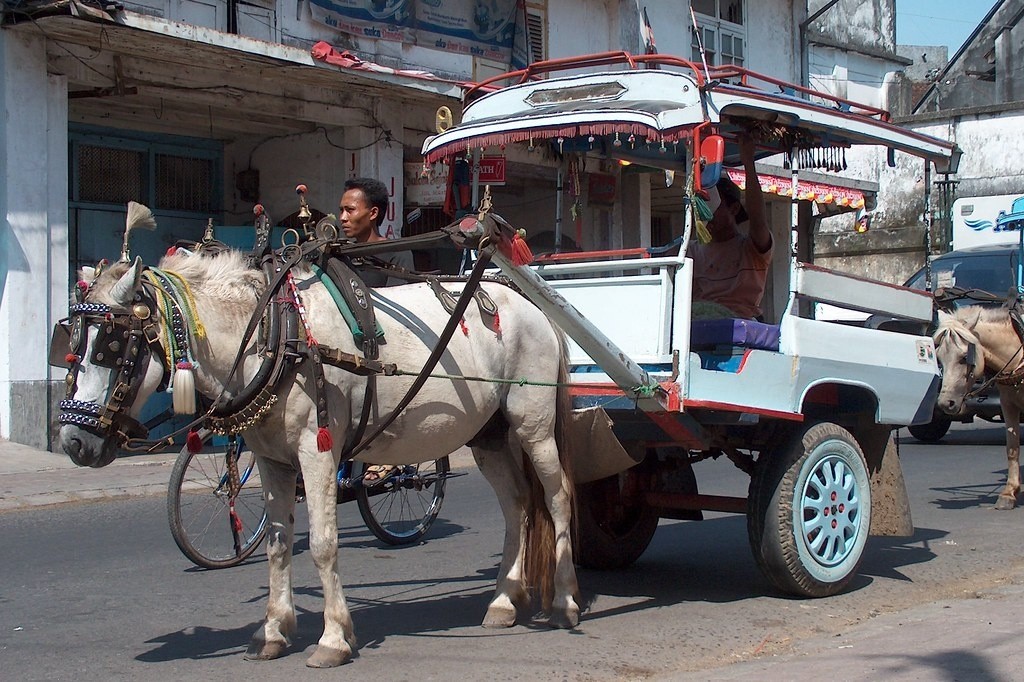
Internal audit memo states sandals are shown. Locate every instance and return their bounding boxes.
[362,464,398,487]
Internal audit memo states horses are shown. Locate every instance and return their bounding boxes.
[931,305,1024,511]
[60,202,579,662]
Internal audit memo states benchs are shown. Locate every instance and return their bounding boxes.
[568,318,779,374]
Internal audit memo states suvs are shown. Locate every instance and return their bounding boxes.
[903,243,1024,439]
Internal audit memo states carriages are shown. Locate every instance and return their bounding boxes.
[57,52,966,668]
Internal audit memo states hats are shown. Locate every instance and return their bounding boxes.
[718,178,749,225]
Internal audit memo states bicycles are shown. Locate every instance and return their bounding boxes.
[168,423,468,569]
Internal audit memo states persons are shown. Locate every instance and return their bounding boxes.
[339,176,416,487]
[952,263,980,289]
[683,133,774,321]
